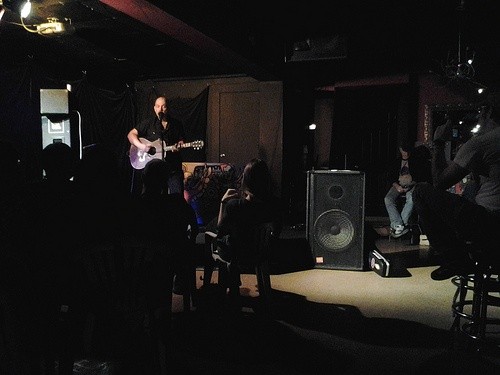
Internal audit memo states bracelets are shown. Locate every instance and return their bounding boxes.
[220,200,224,205]
[433,137,446,148]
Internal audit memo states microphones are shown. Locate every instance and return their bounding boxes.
[158,112,164,119]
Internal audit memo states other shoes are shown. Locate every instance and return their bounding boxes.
[431,263,464,280]
[395,226,404,235]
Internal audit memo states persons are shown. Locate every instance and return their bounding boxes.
[127,97,185,199]
[385,140,423,238]
[422,103,500,281]
[0,143,280,375]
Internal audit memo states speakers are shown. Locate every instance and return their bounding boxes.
[306,169,365,271]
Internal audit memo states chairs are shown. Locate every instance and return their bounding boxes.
[202,215,282,304]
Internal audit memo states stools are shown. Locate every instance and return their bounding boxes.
[451,240,500,375]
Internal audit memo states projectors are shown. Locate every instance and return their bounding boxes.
[37,22,74,37]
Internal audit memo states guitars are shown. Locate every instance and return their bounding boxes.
[128,136,205,170]
